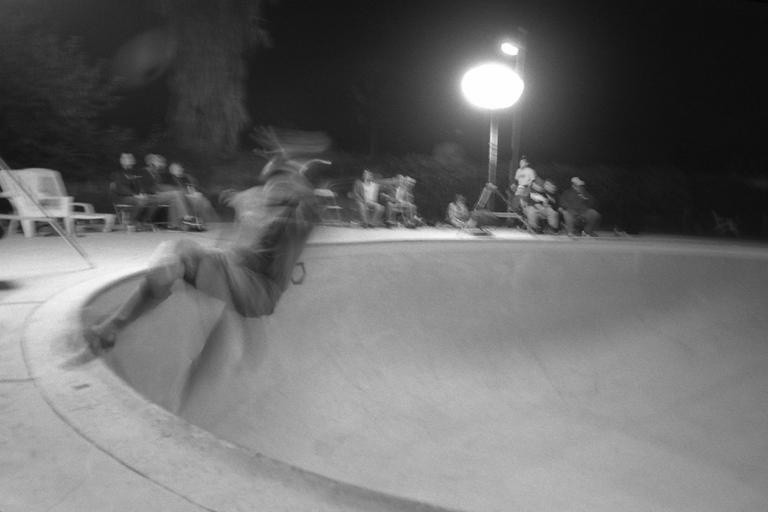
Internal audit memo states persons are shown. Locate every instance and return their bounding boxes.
[76,126,328,352]
[352,169,470,229]
[510,155,601,237]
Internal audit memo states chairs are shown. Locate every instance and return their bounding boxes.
[5,167,201,239]
[312,189,346,227]
